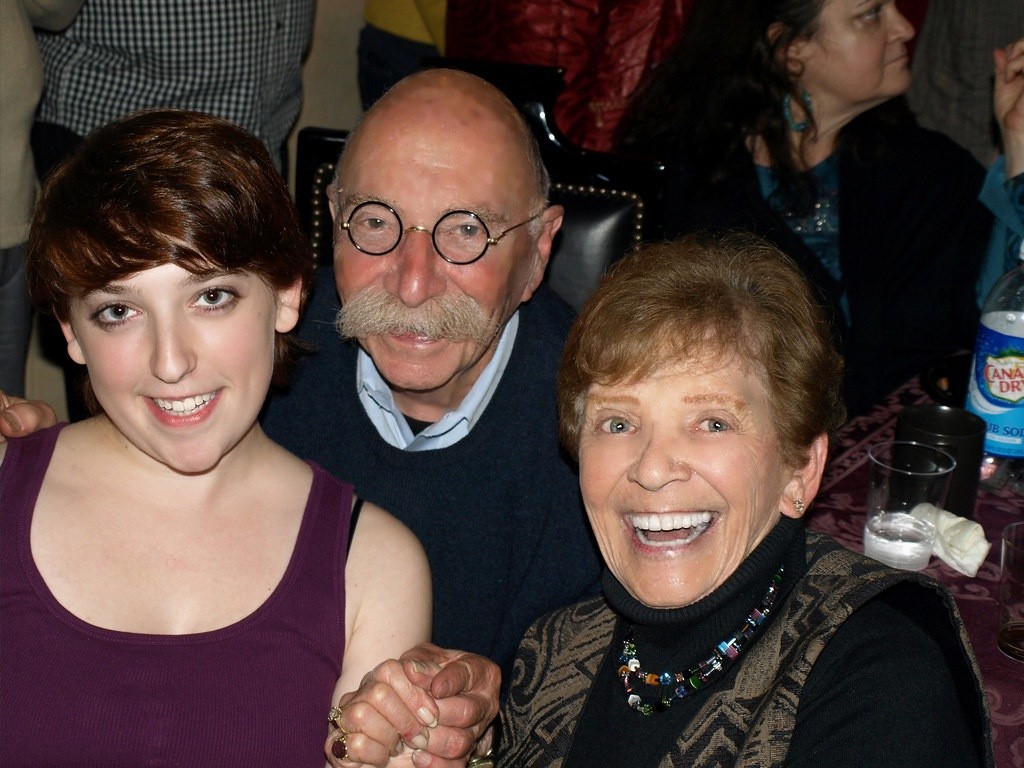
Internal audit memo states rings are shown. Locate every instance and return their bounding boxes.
[329,704,354,735]
[0,109,435,768]
[331,731,350,762]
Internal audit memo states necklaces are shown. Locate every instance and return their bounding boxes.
[604,528,803,714]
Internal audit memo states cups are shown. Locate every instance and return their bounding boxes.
[996,523,1023,667]
[863,441,956,575]
[889,406,988,519]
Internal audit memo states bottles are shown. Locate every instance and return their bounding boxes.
[964,243,1023,497]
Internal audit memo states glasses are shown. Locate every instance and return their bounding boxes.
[336,188,544,265]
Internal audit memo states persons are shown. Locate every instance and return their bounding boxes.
[0,64,615,718]
[355,0,454,143]
[893,1,1023,274]
[616,0,1024,446]
[447,0,694,178]
[0,0,320,270]
[0,4,94,433]
[322,227,991,767]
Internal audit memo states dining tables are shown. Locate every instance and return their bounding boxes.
[801,376,1024,768]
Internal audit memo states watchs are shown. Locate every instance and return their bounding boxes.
[462,740,496,768]
